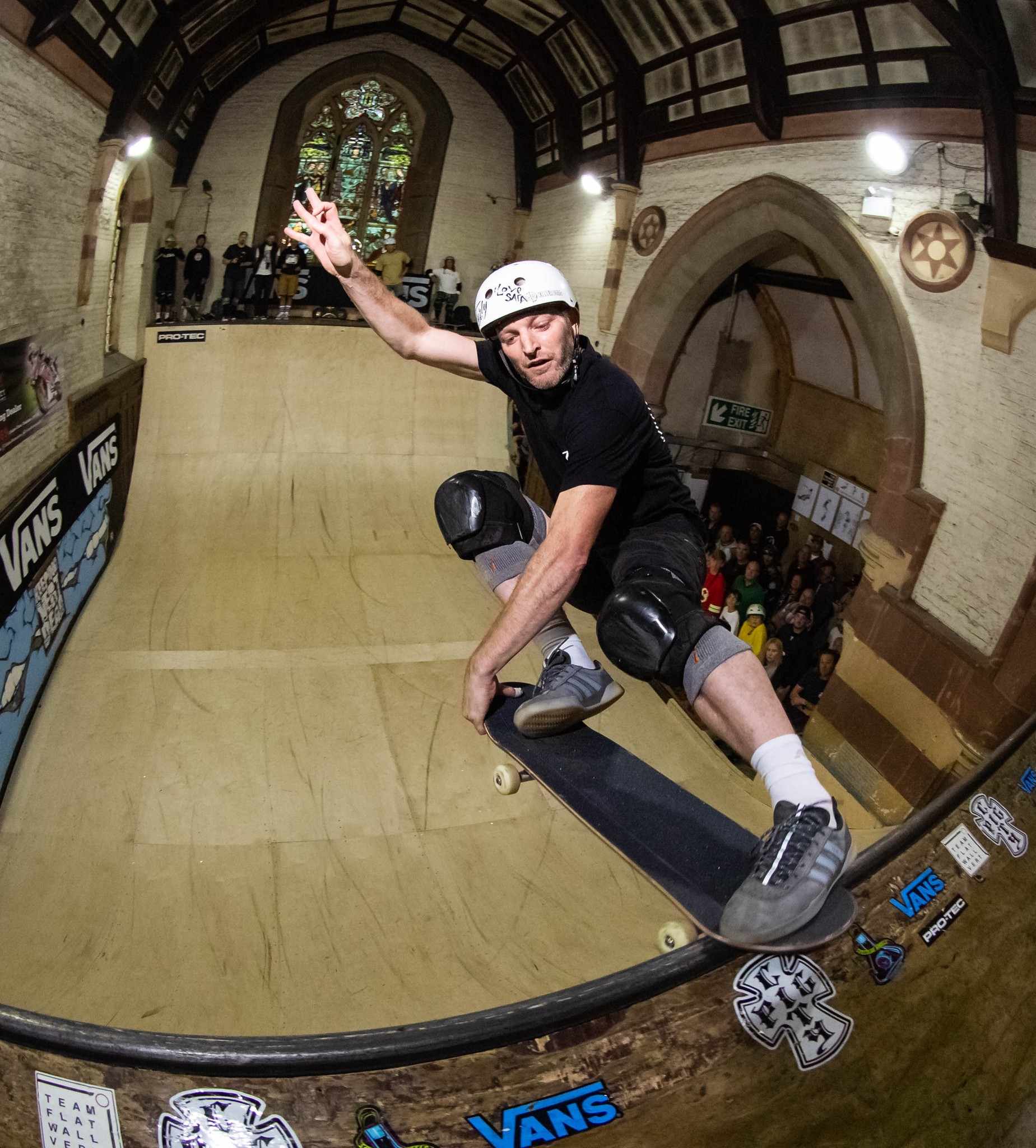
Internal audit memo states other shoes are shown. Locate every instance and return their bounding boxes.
[222,316,236,321]
[253,315,267,320]
[275,312,289,320]
[432,318,440,323]
[444,320,451,324]
[177,316,186,321]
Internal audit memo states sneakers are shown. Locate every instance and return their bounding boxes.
[720,796,858,945]
[163,317,174,323]
[514,647,625,736]
[155,318,163,324]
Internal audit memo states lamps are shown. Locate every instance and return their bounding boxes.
[866,131,945,175]
[581,175,614,196]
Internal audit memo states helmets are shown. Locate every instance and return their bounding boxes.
[745,604,765,619]
[383,238,396,244]
[761,543,778,556]
[475,261,580,342]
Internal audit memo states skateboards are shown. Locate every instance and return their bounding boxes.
[481,680,860,951]
[428,322,467,332]
[181,301,203,320]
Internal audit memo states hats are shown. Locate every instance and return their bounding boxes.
[795,606,811,620]
[446,256,454,260]
[240,231,248,236]
[165,236,176,241]
[846,574,862,589]
[810,535,824,544]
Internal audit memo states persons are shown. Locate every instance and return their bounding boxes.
[693,501,859,739]
[153,230,517,328]
[284,187,854,945]
